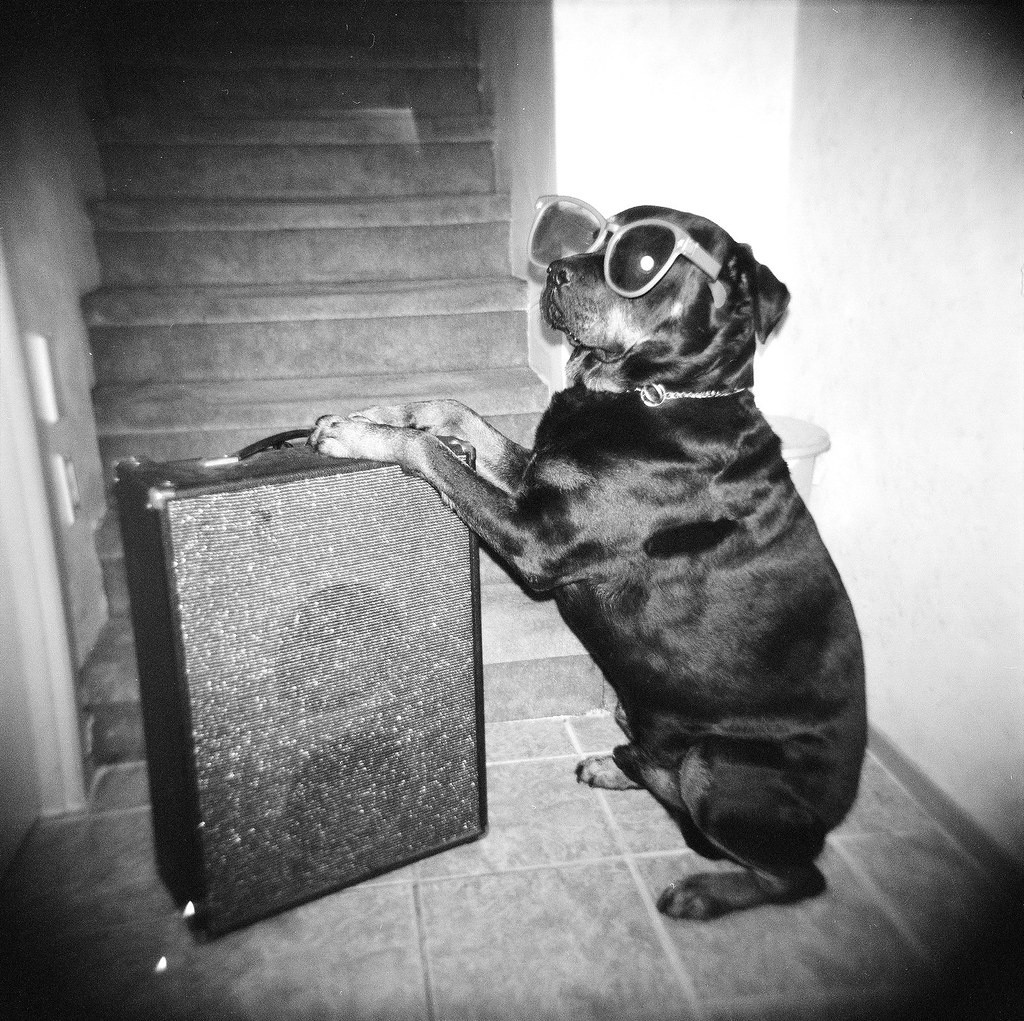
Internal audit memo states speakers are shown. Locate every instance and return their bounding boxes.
[116,433,491,942]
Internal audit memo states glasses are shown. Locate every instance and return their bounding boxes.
[524,193,720,302]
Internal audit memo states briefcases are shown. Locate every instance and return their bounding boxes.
[114,426,489,946]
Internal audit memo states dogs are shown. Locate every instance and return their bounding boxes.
[309,204,869,922]
